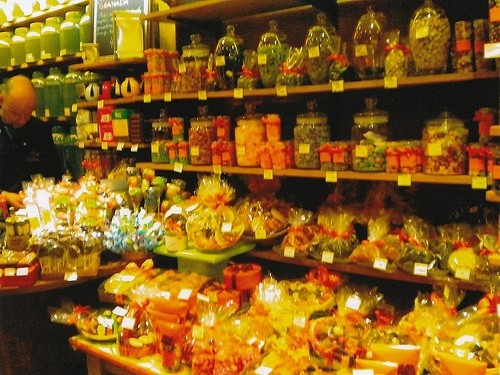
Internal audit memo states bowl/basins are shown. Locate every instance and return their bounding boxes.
[241,211,314,244]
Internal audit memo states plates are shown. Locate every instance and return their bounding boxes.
[80,329,120,340]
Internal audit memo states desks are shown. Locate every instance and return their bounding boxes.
[0,261,129,375]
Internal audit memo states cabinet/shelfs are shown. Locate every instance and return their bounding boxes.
[0,0,500,375]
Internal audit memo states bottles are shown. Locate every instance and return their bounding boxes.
[142,48,179,94]
[151,97,468,175]
[1,0,94,119]
[179,0,451,91]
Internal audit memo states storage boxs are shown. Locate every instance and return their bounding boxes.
[97,106,115,141]
[112,109,131,142]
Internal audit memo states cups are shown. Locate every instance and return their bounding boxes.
[164,230,191,251]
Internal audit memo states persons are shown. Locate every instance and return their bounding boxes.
[0,76,66,210]
